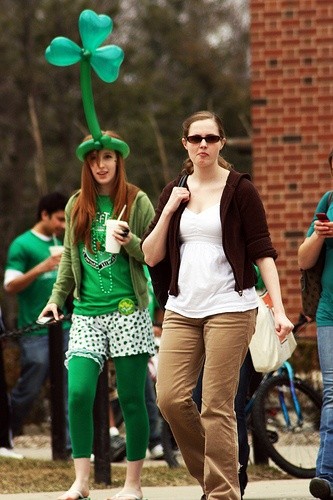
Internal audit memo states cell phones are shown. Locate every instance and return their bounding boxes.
[36,310,63,326]
[316,213,330,223]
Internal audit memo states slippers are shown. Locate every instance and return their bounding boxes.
[106,493,148,500]
[58,491,91,500]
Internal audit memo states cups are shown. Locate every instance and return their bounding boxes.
[105,220,128,254]
[49,246,64,267]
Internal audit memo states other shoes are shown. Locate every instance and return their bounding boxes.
[309,477,333,500]
[149,444,163,460]
[0,447,25,459]
[108,435,127,461]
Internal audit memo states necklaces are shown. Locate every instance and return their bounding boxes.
[92,216,113,293]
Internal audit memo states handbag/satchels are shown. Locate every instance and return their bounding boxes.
[146,174,189,311]
[300,190,333,318]
[248,287,298,373]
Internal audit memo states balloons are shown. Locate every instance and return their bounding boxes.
[45,9,130,160]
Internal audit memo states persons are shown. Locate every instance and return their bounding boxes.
[0,150,333,500]
[139,111,295,500]
[36,130,156,500]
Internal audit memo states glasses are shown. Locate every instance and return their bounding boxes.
[183,135,222,144]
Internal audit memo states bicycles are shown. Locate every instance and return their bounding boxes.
[160,311,323,479]
